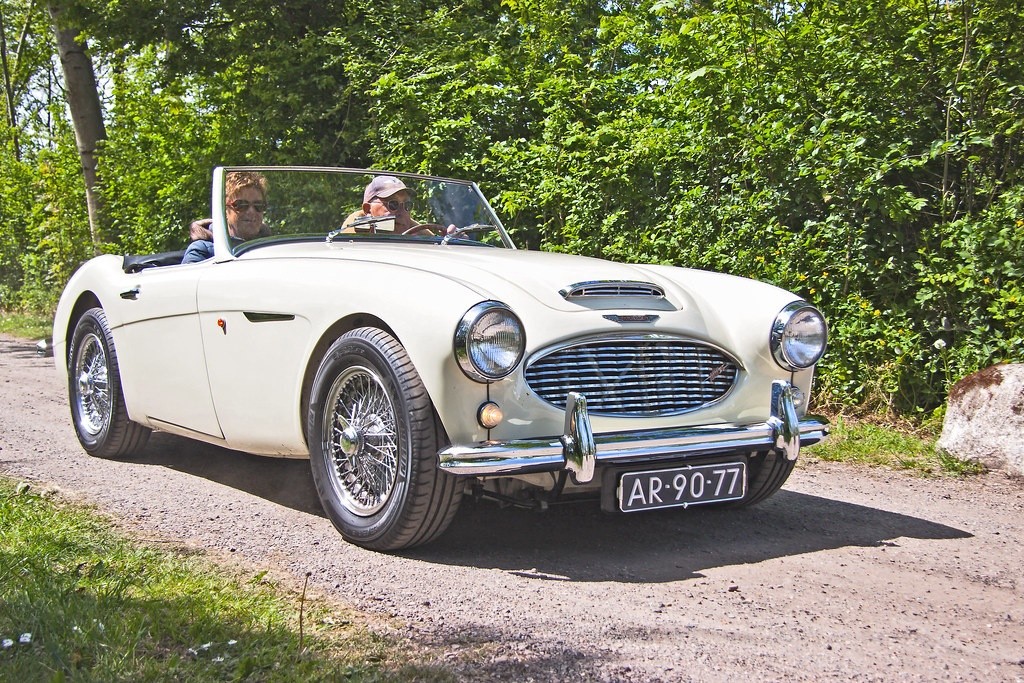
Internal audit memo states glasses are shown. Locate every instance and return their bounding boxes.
[226,199,267,212]
[370,200,413,212]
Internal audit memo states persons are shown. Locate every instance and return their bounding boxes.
[181,171,272,265]
[340,176,469,240]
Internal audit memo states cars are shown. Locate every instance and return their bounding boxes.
[52,166,830,552]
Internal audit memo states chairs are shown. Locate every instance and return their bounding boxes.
[340,208,435,234]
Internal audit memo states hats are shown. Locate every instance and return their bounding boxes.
[363,176,417,202]
